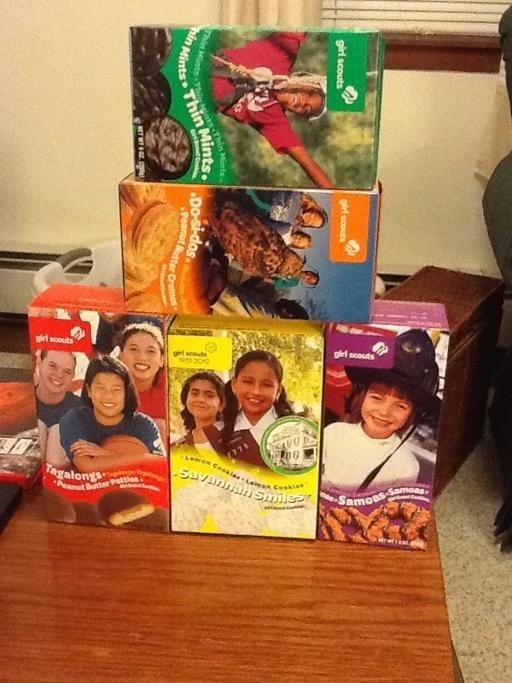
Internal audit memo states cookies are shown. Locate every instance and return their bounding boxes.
[317,502,430,544]
[120,184,178,313]
[41,490,170,533]
[131,26,193,181]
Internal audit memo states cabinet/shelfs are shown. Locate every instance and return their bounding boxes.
[379,267,505,498]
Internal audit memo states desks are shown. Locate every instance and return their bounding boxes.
[0,470,462,681]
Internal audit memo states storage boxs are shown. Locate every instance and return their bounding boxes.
[32,239,385,297]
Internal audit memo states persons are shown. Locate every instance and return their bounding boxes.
[35,311,166,474]
[211,32,338,188]
[200,189,329,319]
[321,364,442,486]
[171,349,319,470]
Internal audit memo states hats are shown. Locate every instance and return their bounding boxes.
[342,329,443,414]
